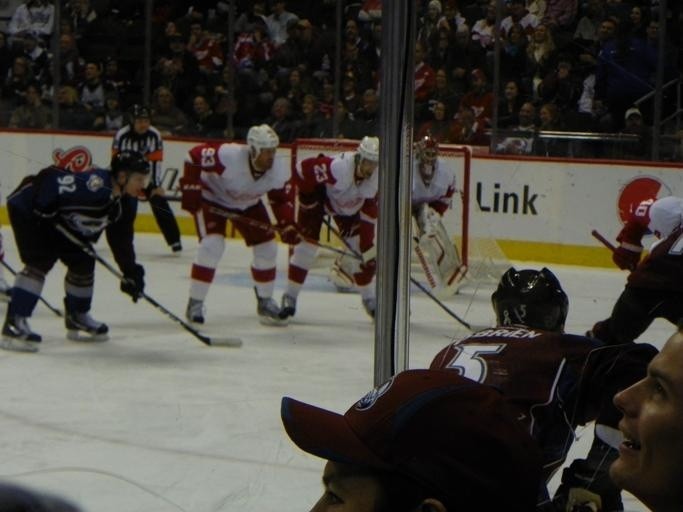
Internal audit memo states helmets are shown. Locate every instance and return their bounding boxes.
[356,135,380,162]
[246,124,280,150]
[111,150,151,176]
[129,104,152,126]
[490,267,568,332]
[414,136,440,165]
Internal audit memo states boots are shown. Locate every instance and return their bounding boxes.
[282,293,298,319]
[62,305,110,342]
[171,240,183,257]
[363,296,376,323]
[256,296,288,326]
[185,296,205,332]
[0,303,42,351]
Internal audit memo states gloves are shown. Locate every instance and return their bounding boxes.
[180,183,204,212]
[614,246,642,271]
[35,211,60,228]
[280,225,303,244]
[121,265,145,304]
[352,261,378,286]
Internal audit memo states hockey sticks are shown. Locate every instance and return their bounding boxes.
[55,220,243,348]
[411,277,491,333]
[1,260,64,319]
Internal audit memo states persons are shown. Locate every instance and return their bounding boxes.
[411,136,473,295]
[1,2,683,160]
[0,150,151,343]
[112,103,183,253]
[609,319,682,512]
[585,197,682,344]
[178,125,302,323]
[428,267,659,512]
[281,136,380,316]
